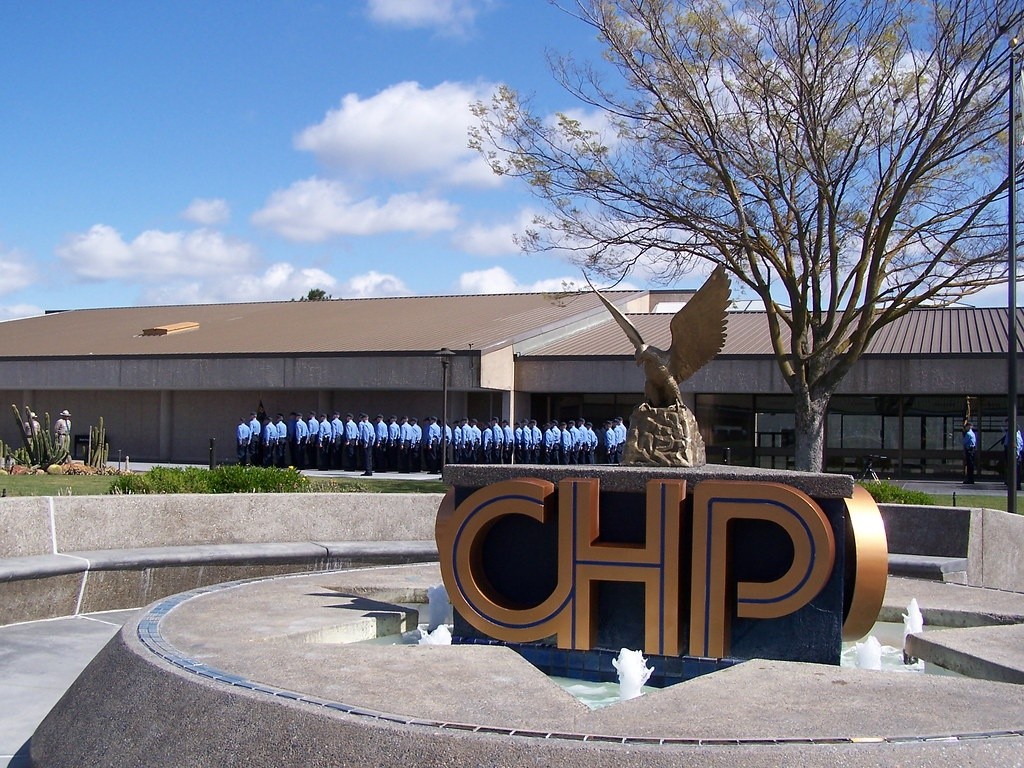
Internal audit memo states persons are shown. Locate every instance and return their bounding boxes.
[233,410,627,479]
[960,422,977,484]
[1016,424,1022,490]
[54,409,71,464]
[1004,418,1021,486]
[23,411,41,452]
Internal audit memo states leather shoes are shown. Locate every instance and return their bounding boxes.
[238,462,442,476]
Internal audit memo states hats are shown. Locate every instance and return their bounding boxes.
[250,412,623,424]
